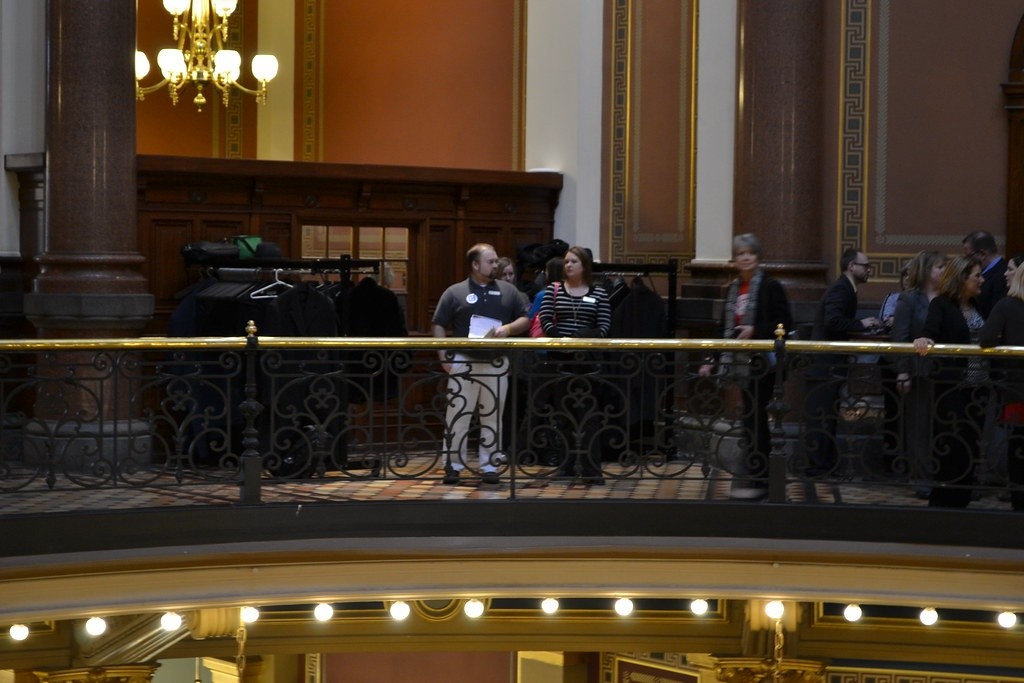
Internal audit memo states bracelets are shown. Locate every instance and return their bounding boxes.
[503,324,510,334]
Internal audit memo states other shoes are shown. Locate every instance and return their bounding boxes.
[996,490,1010,501]
[583,476,605,485]
[483,471,500,484]
[444,471,459,485]
[870,465,901,483]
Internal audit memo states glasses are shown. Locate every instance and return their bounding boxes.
[852,261,870,270]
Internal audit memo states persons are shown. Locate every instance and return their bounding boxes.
[797,248,881,480]
[877,229,1024,511]
[496,239,611,485]
[698,233,792,500]
[430,244,530,485]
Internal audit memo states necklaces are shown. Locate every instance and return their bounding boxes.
[569,288,585,325]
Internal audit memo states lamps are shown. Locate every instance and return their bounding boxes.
[135,0,278,112]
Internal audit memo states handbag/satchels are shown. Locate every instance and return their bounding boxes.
[698,356,718,394]
[529,281,559,338]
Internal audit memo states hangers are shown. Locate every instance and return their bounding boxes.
[193,262,376,300]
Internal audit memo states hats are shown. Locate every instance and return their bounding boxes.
[515,241,543,259]
